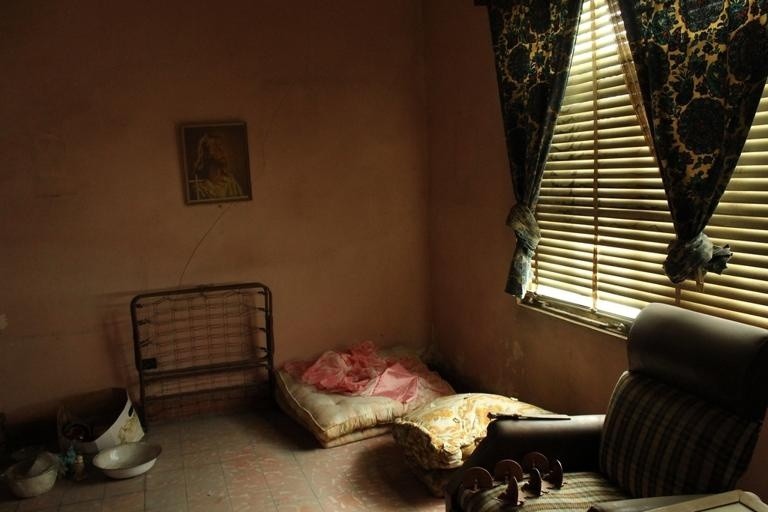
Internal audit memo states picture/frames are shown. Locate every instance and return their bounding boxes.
[178,118,253,206]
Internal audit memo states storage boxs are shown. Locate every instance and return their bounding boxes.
[54,385,147,457]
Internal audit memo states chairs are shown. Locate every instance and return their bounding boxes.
[439,302,768,512]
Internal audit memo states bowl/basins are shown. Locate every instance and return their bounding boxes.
[92,441,163,479]
[6,459,59,498]
